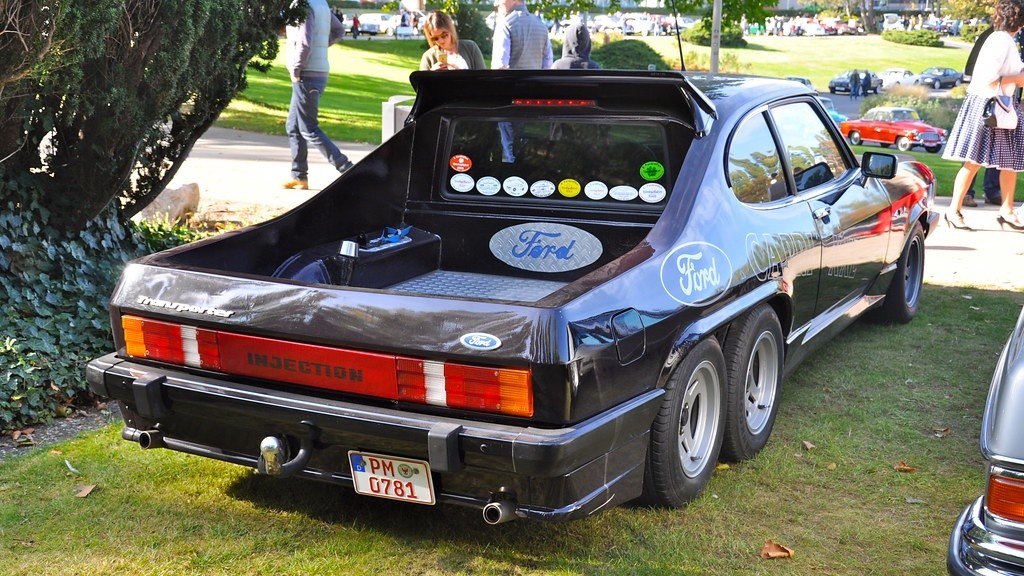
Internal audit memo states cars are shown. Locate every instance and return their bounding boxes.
[828,69,884,95]
[814,95,850,129]
[839,106,948,153]
[784,75,818,92]
[747,14,866,36]
[341,10,429,36]
[535,10,705,40]
[880,12,985,35]
[945,310,1024,576]
[919,67,963,90]
[879,69,921,91]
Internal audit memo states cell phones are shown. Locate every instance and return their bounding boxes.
[439,54,448,67]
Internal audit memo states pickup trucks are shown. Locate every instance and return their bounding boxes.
[84,68,939,519]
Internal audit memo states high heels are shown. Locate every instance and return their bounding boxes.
[944,211,970,231]
[996,214,1024,231]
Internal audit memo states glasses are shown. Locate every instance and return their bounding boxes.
[430,32,450,42]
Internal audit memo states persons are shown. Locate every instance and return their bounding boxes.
[281,0,346,189]
[551,22,611,149]
[849,69,870,100]
[740,14,802,36]
[621,12,671,37]
[331,4,343,24]
[419,9,485,70]
[489,0,553,162]
[903,13,971,38]
[941,0,1024,231]
[353,14,361,39]
[400,8,418,39]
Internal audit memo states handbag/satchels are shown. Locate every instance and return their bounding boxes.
[983,95,1018,130]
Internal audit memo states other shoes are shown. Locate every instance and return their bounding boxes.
[986,194,1001,206]
[284,180,309,189]
[962,195,978,206]
